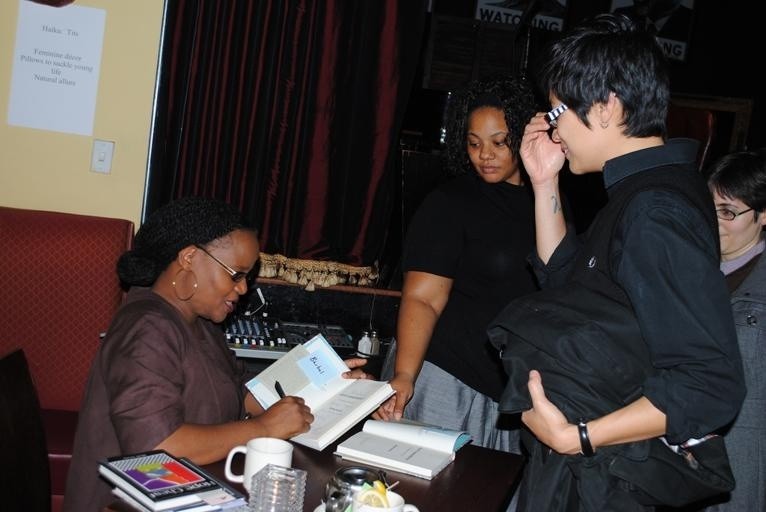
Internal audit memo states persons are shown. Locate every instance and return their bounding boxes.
[58,193,315,511]
[368,92,533,512]
[520,10,749,511]
[701,146,765,512]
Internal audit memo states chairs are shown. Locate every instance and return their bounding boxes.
[0,349,51,512]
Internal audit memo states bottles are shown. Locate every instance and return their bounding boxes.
[356,330,381,358]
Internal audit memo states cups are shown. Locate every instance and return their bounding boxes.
[351,488,408,511]
[222,435,294,490]
[321,467,391,510]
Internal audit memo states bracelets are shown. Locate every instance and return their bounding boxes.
[577,414,595,458]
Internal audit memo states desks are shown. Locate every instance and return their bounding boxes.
[103,421,527,512]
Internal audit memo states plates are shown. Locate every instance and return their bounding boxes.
[312,499,422,511]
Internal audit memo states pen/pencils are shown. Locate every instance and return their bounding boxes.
[273,380,288,400]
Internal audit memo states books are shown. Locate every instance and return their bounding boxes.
[97,449,248,511]
[334,417,474,480]
[244,333,397,452]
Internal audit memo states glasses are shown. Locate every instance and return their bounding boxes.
[544,104,567,128]
[716,207,751,222]
[194,243,247,283]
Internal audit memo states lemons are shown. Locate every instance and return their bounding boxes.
[361,480,388,507]
